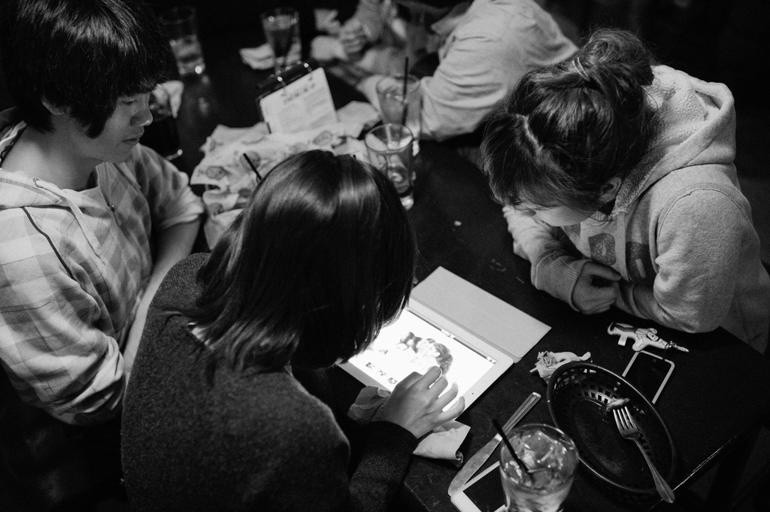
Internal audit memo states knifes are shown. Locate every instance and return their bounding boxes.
[447,389,544,496]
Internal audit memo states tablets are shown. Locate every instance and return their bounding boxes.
[327,300,516,421]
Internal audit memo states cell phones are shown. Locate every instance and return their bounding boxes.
[450,460,505,511]
[607,349,675,410]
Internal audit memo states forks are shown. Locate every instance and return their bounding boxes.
[610,406,676,504]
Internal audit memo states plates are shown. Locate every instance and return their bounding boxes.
[546,359,677,497]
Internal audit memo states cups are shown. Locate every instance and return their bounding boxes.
[500,422,578,512]
[262,7,304,76]
[142,85,185,163]
[156,5,207,78]
[361,71,426,200]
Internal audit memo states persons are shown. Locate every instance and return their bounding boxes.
[338,0,579,146]
[479,29,770,359]
[121,150,466,512]
[1,1,200,425]
